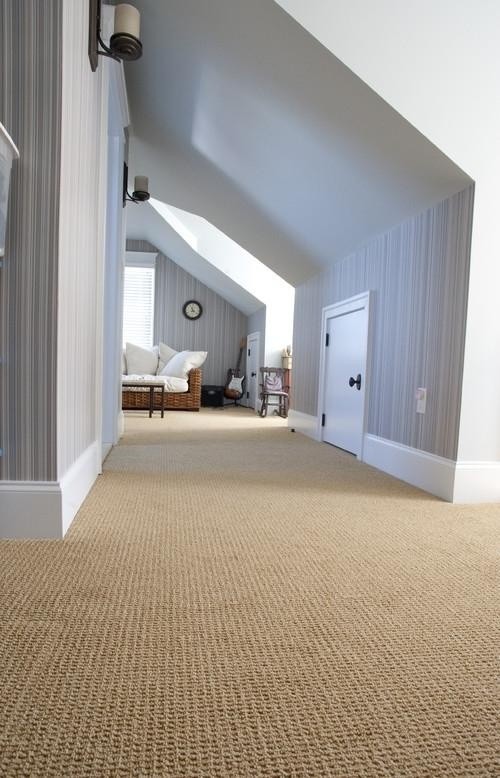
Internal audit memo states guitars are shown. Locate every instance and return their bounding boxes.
[224,335,246,400]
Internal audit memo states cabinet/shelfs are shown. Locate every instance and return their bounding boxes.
[201,384,224,408]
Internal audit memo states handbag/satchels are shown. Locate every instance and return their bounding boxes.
[265,377,282,390]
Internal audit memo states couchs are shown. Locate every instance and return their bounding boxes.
[121,338,208,409]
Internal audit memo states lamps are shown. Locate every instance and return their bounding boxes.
[122,161,151,209]
[88,0,145,72]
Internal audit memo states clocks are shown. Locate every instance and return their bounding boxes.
[183,299,202,320]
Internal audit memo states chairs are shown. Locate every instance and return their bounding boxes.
[259,366,290,418]
[223,367,243,407]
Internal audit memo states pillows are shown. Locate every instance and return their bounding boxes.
[264,374,282,392]
[121,341,207,379]
[228,374,246,393]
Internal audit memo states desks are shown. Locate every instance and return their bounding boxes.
[122,383,165,418]
